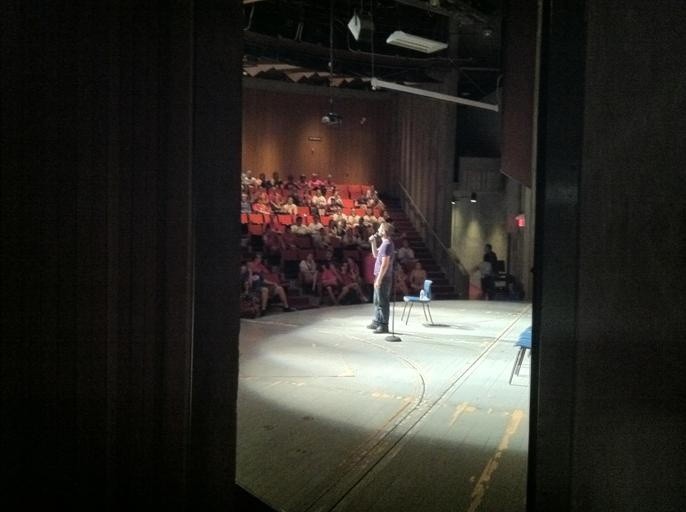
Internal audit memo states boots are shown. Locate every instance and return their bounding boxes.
[366,320,388,333]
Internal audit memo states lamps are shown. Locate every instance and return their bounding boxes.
[320,2,345,124]
[452,196,455,205]
[470,190,477,203]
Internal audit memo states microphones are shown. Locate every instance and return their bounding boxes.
[367,232,380,243]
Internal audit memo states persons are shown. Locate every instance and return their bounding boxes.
[366,223,396,333]
[468,244,499,300]
[242,170,427,320]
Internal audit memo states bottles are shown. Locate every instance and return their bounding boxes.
[420,288,425,300]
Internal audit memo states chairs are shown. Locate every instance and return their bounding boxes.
[509,326,533,384]
[401,280,434,326]
[240,178,382,305]
